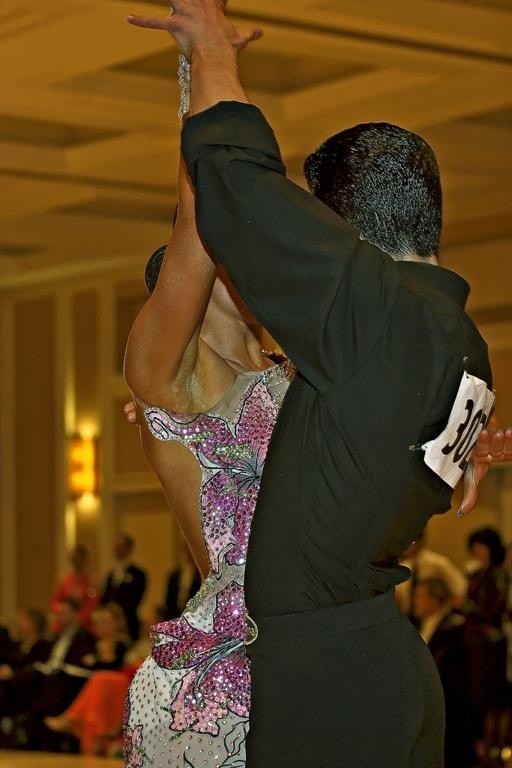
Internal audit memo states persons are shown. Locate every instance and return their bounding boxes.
[393,527,511,768]
[1,534,201,759]
